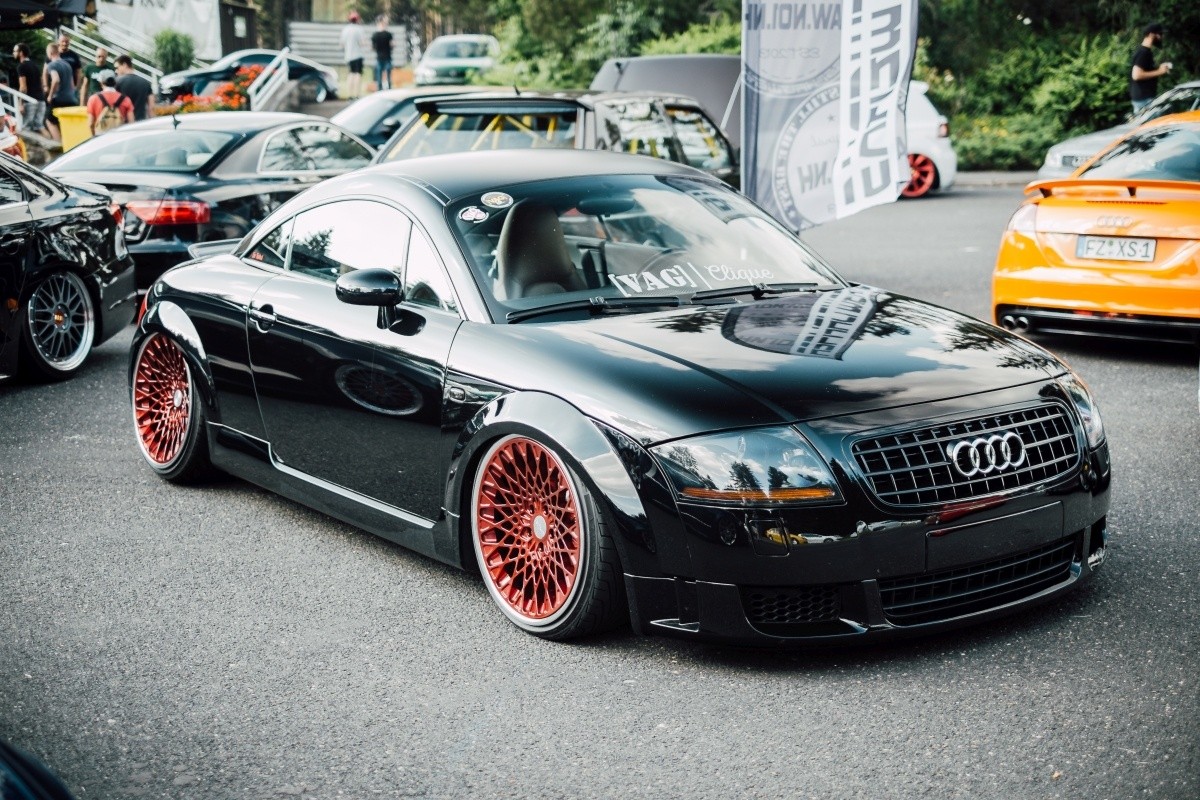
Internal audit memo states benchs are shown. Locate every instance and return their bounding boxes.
[101,149,188,167]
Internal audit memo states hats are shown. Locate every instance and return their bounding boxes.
[96,70,116,83]
[1143,23,1162,35]
[349,12,360,20]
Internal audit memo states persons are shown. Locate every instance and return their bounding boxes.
[371,16,395,91]
[0,35,155,168]
[1130,23,1173,116]
[340,11,369,100]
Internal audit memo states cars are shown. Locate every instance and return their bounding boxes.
[896,79,960,200]
[157,48,342,105]
[993,79,1200,348]
[127,87,1111,652]
[411,32,530,88]
[0,111,381,388]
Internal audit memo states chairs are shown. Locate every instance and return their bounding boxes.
[488,198,587,300]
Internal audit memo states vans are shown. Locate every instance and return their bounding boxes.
[589,55,745,182]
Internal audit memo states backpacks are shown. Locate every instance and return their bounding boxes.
[95,90,127,135]
